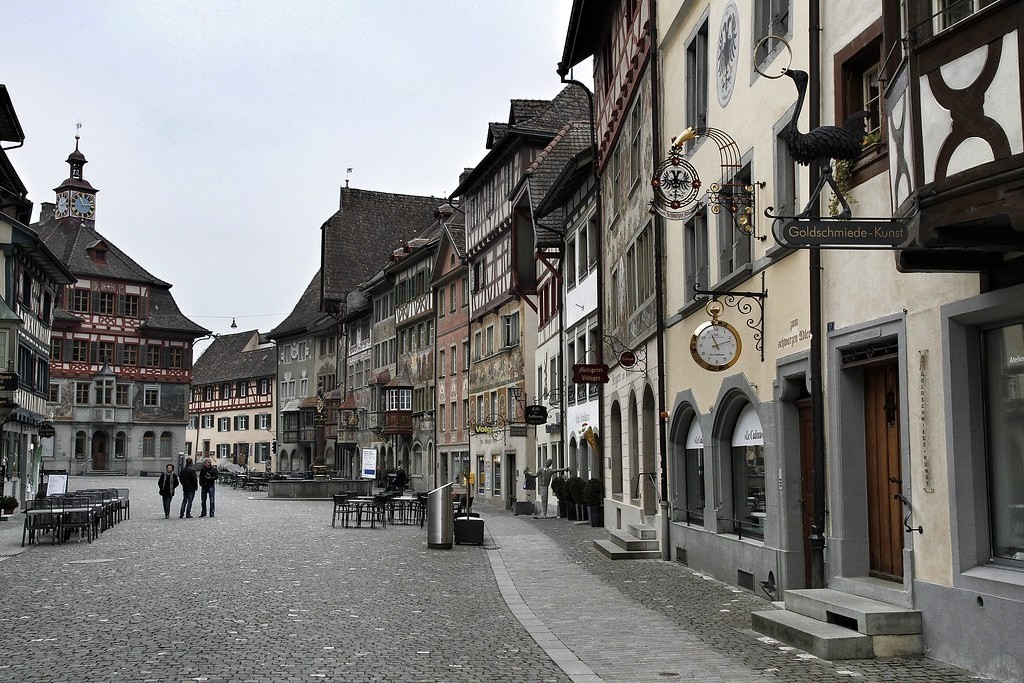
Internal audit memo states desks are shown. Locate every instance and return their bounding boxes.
[45,504,103,538]
[90,498,118,527]
[27,508,93,545]
[218,472,234,485]
[347,499,375,528]
[356,495,379,521]
[390,497,418,525]
[287,477,306,480]
[453,502,461,513]
[250,477,267,492]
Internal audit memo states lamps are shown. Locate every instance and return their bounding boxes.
[231,317,237,329]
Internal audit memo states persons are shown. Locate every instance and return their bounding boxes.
[199,458,218,518]
[179,457,199,518]
[396,466,405,497]
[159,463,179,518]
[525,458,570,517]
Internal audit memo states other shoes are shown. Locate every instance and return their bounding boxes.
[198,513,206,518]
[186,515,193,518]
[165,514,170,519]
[179,515,183,518]
[210,514,214,517]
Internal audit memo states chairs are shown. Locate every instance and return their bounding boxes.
[20,487,130,548]
[217,471,306,492]
[331,490,474,530]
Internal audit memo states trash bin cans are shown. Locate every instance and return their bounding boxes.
[427,482,453,549]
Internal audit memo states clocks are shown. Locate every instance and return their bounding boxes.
[689,300,743,372]
[54,189,69,220]
[71,190,97,221]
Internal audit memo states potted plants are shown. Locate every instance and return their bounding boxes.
[0,495,21,515]
[549,474,606,528]
[455,468,485,546]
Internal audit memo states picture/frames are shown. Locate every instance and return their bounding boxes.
[453,513,480,521]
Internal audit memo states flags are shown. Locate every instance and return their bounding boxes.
[77,123,83,128]
[347,168,352,172]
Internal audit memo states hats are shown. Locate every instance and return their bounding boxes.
[397,466,403,470]
